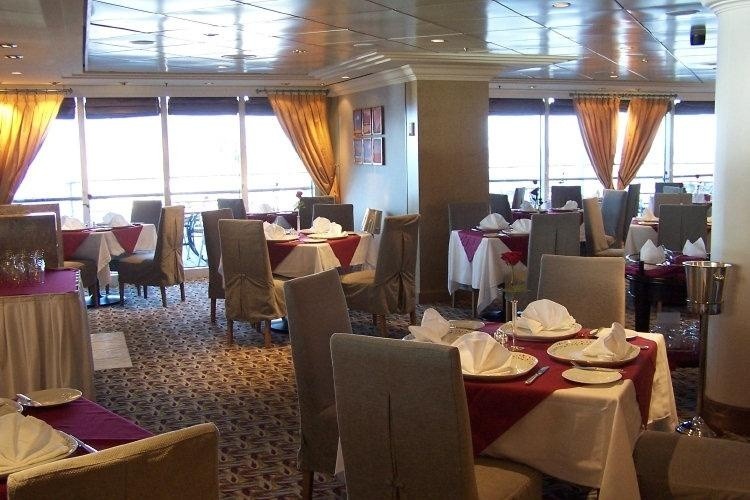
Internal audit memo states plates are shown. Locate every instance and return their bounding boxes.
[471,223,531,237]
[402,327,493,346]
[265,228,359,242]
[93,223,140,231]
[563,366,622,385]
[547,338,640,366]
[590,327,639,340]
[449,319,485,330]
[16,387,83,407]
[462,351,540,377]
[56,429,79,457]
[0,397,23,414]
[513,206,581,214]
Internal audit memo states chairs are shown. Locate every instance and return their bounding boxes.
[1,203,62,227]
[0,212,100,309]
[6,422,220,499]
[107,182,750,499]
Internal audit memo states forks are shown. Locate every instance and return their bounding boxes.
[570,359,623,373]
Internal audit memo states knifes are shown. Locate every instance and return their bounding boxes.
[525,365,550,385]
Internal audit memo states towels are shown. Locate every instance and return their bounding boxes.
[0,413,79,478]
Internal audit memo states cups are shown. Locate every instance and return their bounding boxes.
[0,249,47,287]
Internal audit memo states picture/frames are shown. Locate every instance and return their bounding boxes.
[353,105,384,166]
